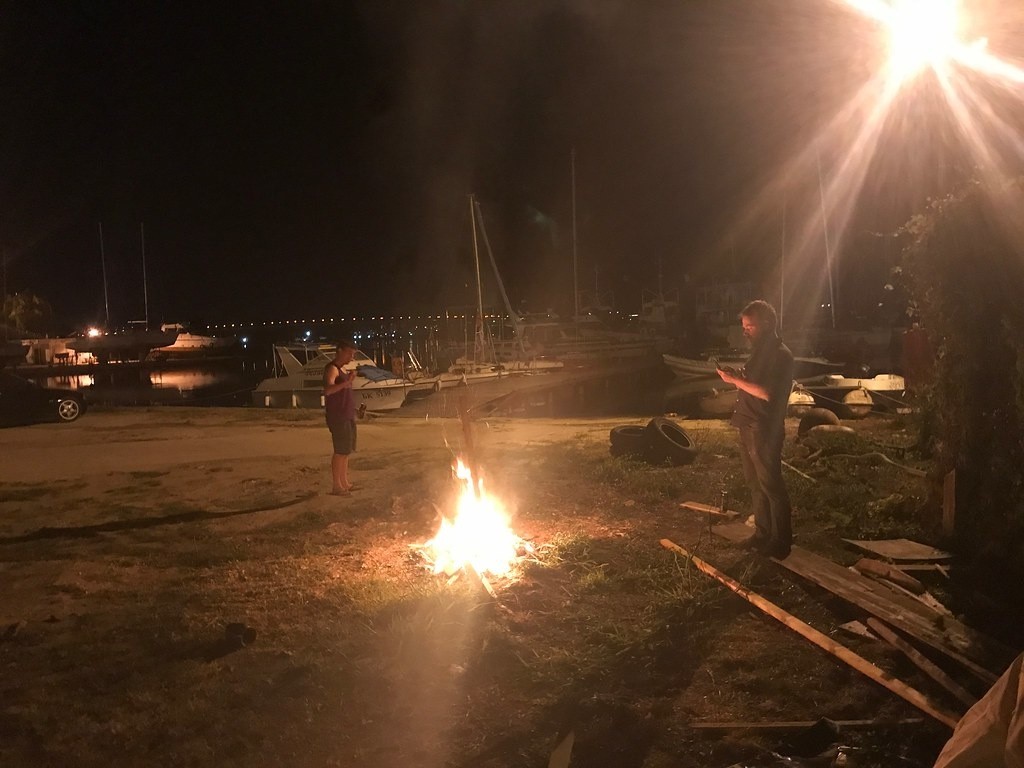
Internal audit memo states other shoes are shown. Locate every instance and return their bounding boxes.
[732,534,767,548]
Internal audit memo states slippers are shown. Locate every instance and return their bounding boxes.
[756,539,792,558]
[328,489,352,498]
[346,485,364,490]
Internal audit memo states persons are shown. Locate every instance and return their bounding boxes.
[322,337,367,499]
[712,299,796,559]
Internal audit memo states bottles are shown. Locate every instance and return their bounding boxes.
[720,490,728,513]
[358,403,366,419]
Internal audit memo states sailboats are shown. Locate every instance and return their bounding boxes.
[470,144,901,394]
[388,188,564,393]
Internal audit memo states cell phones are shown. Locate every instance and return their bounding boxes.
[712,359,728,372]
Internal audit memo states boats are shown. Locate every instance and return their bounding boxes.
[253,331,413,410]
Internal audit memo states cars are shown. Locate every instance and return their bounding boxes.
[0,371,86,427]
